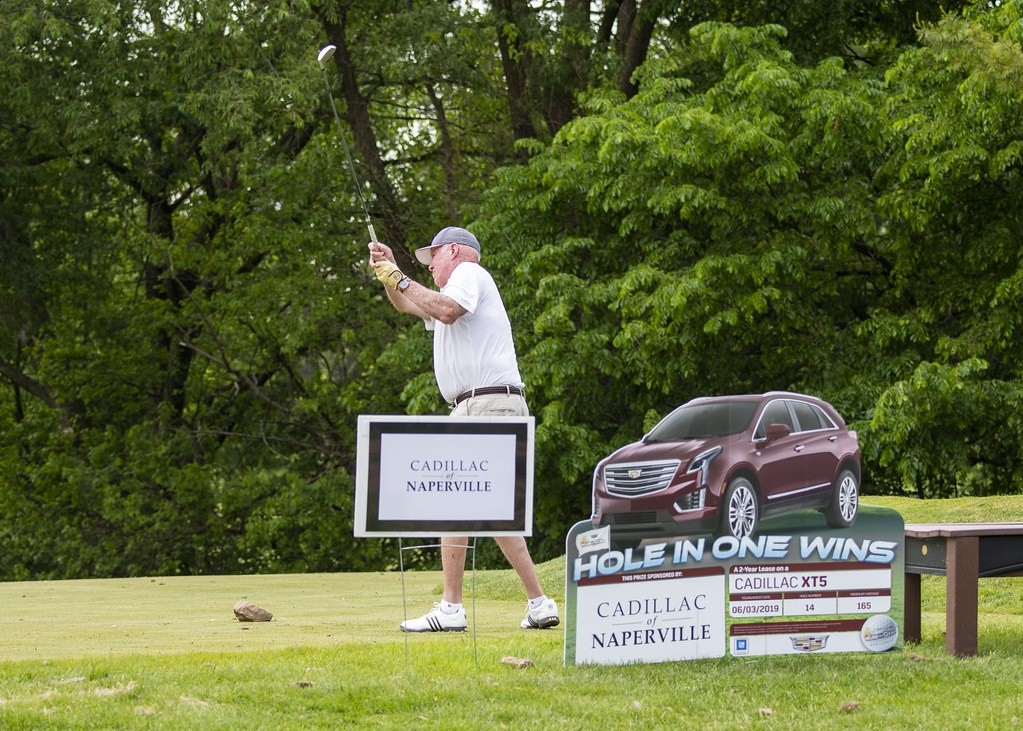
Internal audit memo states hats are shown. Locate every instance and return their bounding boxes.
[414,227,481,266]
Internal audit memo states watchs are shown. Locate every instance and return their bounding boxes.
[399,279,411,293]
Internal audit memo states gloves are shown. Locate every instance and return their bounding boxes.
[373,259,407,290]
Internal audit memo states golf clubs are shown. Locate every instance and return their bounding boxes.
[316,44,381,252]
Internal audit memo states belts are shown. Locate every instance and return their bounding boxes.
[449,385,525,408]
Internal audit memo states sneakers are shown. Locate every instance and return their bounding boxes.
[401,602,467,633]
[520,600,559,629]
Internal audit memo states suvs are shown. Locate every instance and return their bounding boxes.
[591,388,863,553]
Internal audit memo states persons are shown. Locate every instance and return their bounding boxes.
[366,227,560,632]
